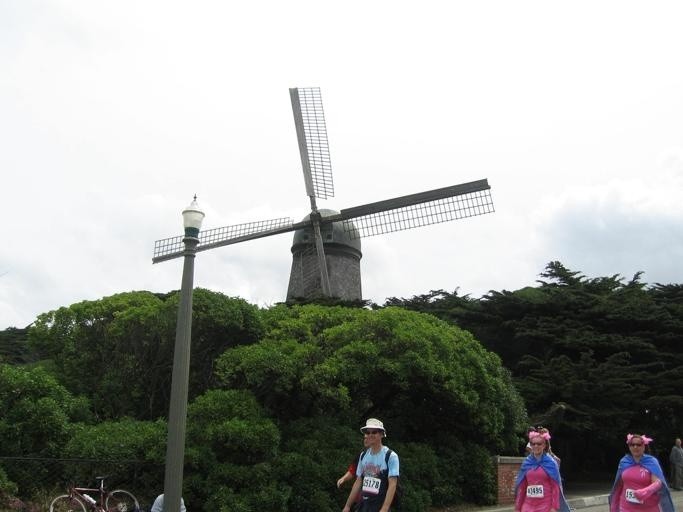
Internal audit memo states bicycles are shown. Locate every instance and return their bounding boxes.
[49,476,140,512]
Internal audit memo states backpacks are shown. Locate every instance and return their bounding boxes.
[361,449,404,506]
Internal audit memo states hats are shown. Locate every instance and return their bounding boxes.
[360,418,385,434]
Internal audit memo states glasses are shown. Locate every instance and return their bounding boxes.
[631,444,642,446]
[532,442,544,445]
[365,430,382,434]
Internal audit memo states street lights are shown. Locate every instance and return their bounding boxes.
[162,194,204,512]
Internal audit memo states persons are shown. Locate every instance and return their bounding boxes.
[335,433,369,490]
[150,493,187,512]
[610,432,675,511]
[513,435,572,511]
[341,418,403,511]
[524,424,560,467]
[668,437,682,490]
